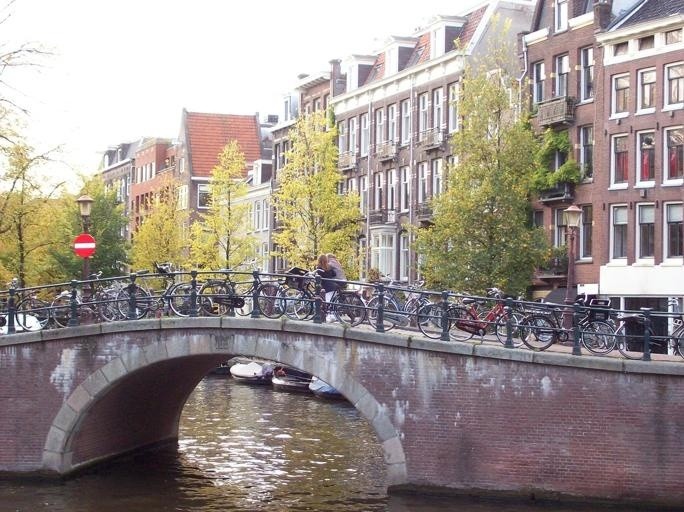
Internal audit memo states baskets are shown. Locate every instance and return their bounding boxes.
[286,267,309,290]
[589,299,611,321]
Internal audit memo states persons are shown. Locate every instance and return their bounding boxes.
[325,253,356,324]
[312,255,338,293]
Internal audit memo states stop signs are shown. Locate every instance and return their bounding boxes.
[73,233,96,258]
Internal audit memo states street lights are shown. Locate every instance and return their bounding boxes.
[75,193,96,300]
[561,203,585,347]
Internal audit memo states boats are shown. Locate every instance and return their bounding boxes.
[212,355,350,404]
